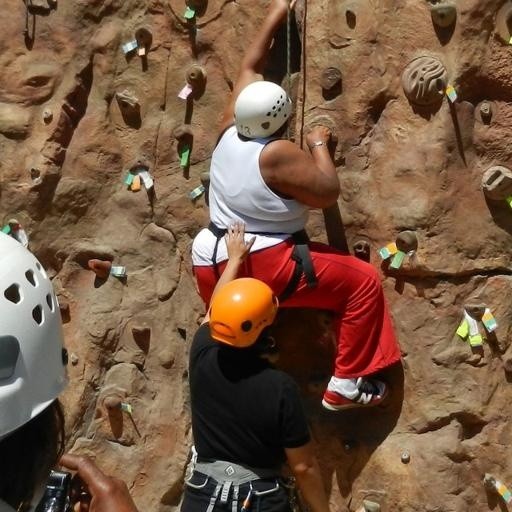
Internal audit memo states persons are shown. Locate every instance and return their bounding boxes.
[178,220,330,512]
[190,1,404,412]
[0,226,142,512]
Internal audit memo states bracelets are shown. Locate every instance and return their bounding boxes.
[309,141,327,149]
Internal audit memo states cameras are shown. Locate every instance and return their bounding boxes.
[34,469,72,512]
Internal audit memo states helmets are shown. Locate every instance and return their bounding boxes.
[209,277,279,347]
[234,81,292,139]
[0,231,69,438]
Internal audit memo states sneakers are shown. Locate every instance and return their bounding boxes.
[322,375,389,411]
